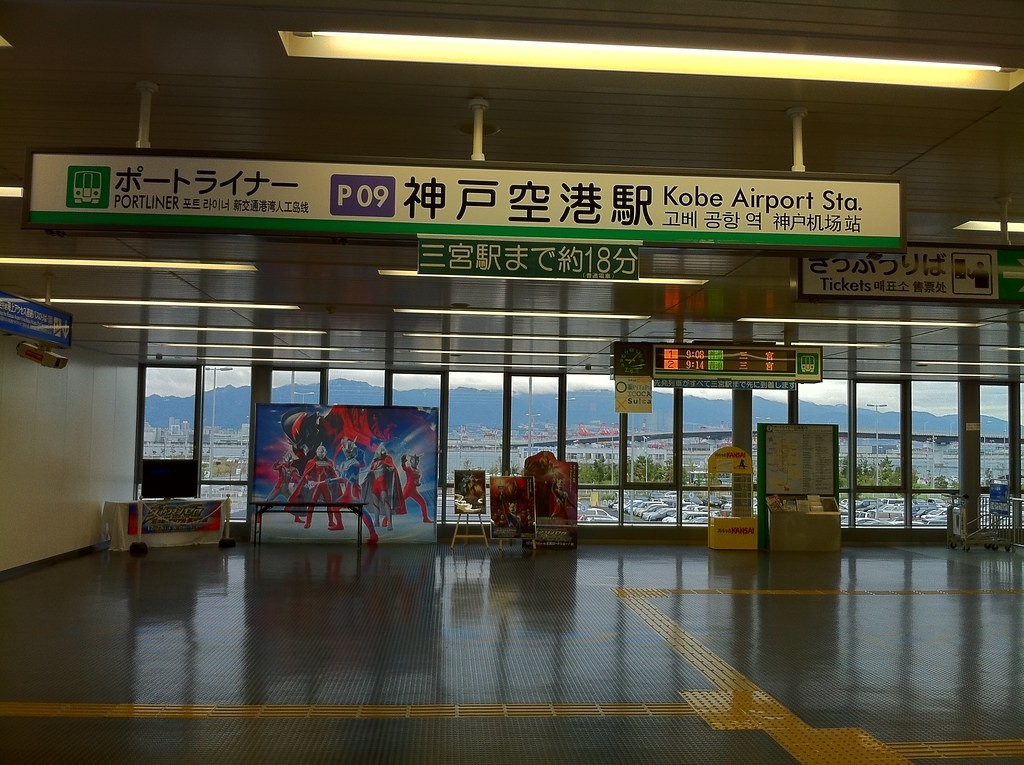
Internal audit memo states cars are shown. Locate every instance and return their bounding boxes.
[839,494,1024,524]
[578,493,758,524]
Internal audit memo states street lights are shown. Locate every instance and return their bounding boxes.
[526,413,541,456]
[867,404,887,487]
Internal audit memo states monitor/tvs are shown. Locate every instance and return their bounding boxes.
[141,459,198,501]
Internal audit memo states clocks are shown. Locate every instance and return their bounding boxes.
[613,342,653,379]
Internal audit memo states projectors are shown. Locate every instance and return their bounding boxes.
[16,343,68,370]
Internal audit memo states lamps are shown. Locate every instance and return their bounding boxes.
[290,31,1019,72]
